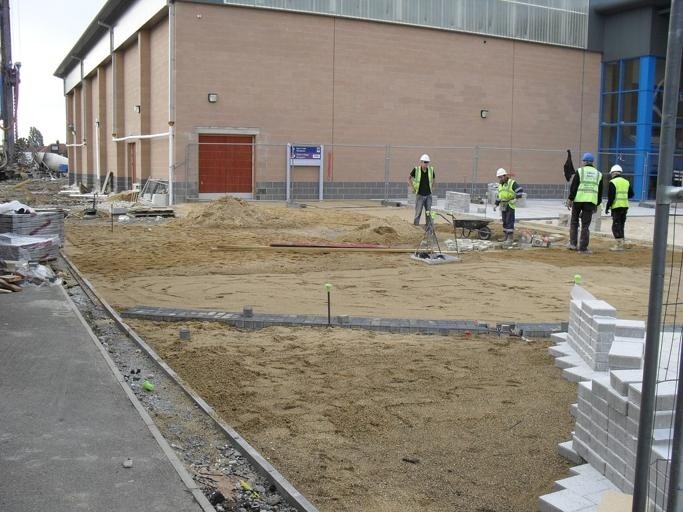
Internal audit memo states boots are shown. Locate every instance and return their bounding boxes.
[608,238,625,251]
[497,228,514,243]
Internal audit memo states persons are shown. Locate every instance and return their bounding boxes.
[406,153,436,229]
[493,167,524,242]
[605,163,635,251]
[565,152,603,254]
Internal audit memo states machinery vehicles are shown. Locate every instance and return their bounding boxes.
[18,151,69,172]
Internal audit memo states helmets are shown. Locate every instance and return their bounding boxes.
[496,168,507,177]
[581,153,594,161]
[609,165,622,174]
[420,154,431,161]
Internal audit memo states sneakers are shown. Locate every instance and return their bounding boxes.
[566,241,592,254]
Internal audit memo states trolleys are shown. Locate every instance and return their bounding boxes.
[436,213,494,240]
